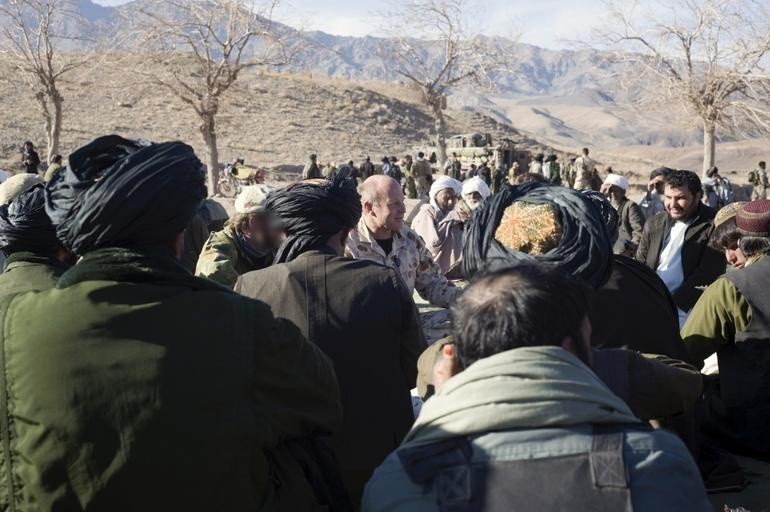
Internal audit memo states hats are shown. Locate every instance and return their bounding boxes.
[714,202,748,227]
[235,185,275,213]
[736,200,770,232]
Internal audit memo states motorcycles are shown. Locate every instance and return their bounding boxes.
[215,154,268,200]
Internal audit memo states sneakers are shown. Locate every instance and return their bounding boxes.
[700,467,745,494]
